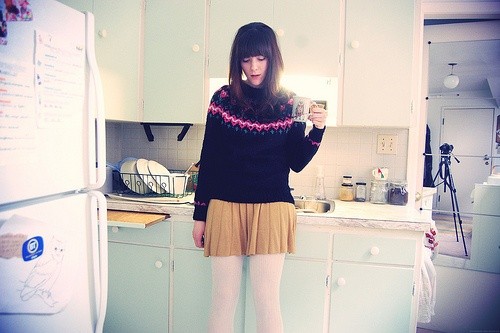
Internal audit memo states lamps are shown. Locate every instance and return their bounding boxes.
[444,63,459,89]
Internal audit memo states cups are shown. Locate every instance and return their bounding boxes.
[292,96,318,122]
[172,173,187,194]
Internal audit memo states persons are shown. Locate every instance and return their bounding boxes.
[0,0,32,38]
[192,23,327,333]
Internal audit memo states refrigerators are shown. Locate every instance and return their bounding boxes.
[0,0,108,333]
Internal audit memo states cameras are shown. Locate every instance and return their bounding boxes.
[440,143,453,154]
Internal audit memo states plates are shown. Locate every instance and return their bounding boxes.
[120,161,148,194]
[148,160,173,194]
[137,159,165,193]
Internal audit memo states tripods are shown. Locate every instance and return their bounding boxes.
[430,153,468,256]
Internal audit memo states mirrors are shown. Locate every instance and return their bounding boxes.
[416,39,500,333]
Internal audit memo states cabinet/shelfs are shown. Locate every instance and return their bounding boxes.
[101,210,430,333]
[55,0,417,131]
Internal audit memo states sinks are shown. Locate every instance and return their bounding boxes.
[294,199,336,217]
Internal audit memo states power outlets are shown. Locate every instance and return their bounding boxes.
[377,134,398,155]
